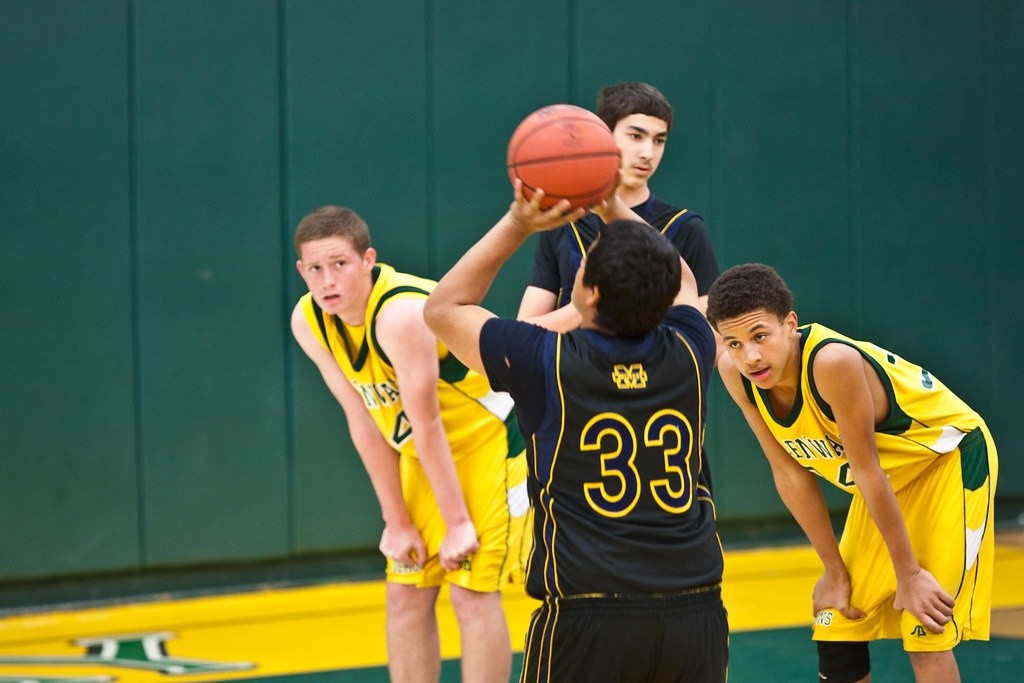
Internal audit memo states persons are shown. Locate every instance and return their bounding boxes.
[423,147,729,683]
[516,84,718,334]
[705,264,999,683]
[291,208,533,683]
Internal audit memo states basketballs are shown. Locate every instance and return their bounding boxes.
[504,102,621,216]
